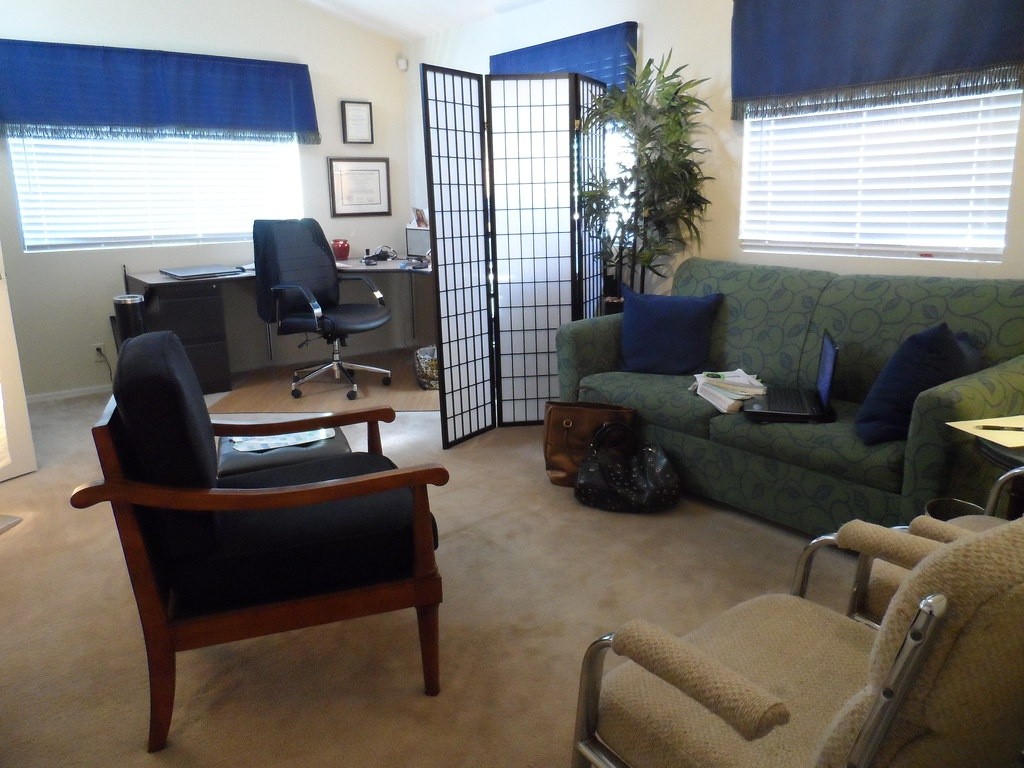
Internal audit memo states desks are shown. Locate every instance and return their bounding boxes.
[129,250,433,383]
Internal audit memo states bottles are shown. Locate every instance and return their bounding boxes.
[331,239,350,261]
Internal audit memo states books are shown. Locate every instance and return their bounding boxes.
[696,368,765,411]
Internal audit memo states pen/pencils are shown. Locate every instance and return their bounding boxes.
[976,424,1022,433]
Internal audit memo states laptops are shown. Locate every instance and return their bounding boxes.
[159,266,242,280]
[744,328,839,424]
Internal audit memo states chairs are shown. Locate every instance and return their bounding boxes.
[67,325,453,752]
[574,513,1024,768]
[251,216,394,398]
[845,465,1024,629]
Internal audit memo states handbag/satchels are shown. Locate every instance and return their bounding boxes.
[574,421,680,513]
[543,385,635,485]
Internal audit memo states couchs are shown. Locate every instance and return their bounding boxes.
[556,252,1024,530]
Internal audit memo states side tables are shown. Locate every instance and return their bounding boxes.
[972,417,1024,521]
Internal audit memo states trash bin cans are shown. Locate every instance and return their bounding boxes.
[414,346,439,391]
[925,495,987,522]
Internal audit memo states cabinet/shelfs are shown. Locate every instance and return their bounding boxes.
[125,269,232,396]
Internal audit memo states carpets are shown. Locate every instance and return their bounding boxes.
[200,344,439,415]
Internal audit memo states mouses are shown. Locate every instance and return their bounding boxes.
[235,267,246,272]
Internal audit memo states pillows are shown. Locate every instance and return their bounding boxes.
[853,322,976,446]
[619,282,723,373]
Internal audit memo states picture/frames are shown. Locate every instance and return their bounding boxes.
[341,101,375,144]
[326,156,392,217]
